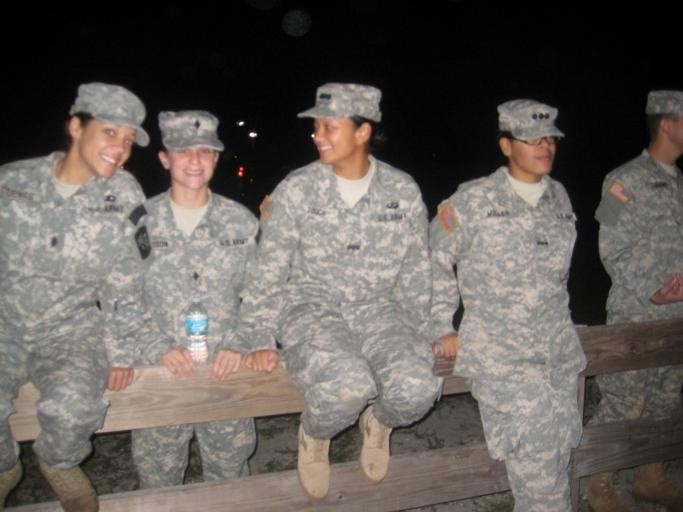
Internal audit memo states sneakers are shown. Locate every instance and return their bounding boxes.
[0,457,24,507]
[297,422,332,499]
[358,405,394,481]
[39,454,99,512]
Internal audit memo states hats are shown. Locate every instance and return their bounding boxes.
[70,83,150,148]
[297,82,383,124]
[159,110,225,152]
[497,99,566,141]
[646,91,683,114]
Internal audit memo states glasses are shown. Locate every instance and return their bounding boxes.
[508,134,560,145]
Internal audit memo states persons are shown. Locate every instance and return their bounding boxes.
[426,98,587,512]
[0,82,154,511]
[123,109,261,489]
[244,82,437,500]
[577,89,682,512]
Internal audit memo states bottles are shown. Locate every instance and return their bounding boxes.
[183,297,209,365]
